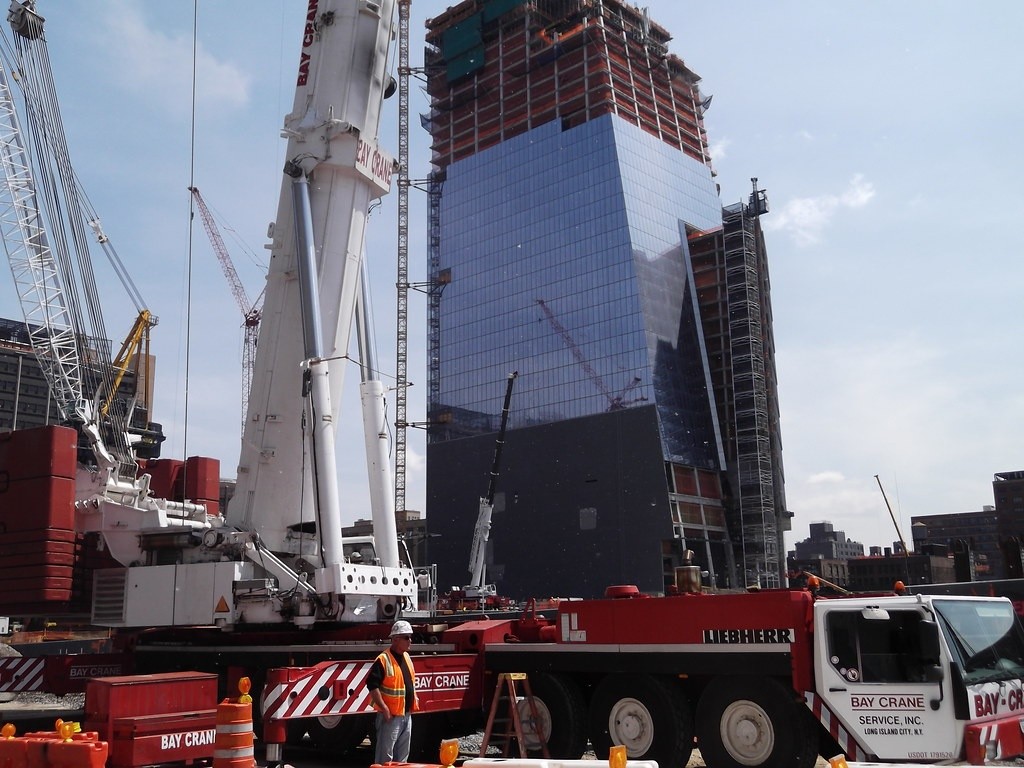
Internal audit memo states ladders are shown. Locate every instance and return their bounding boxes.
[479,672,549,759]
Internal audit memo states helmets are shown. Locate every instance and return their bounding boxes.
[388,621,413,638]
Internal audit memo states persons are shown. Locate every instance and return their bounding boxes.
[366,620,419,764]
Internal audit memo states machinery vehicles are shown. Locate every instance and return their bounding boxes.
[0,0,1024,768]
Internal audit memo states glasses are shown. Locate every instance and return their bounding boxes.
[398,636,413,641]
